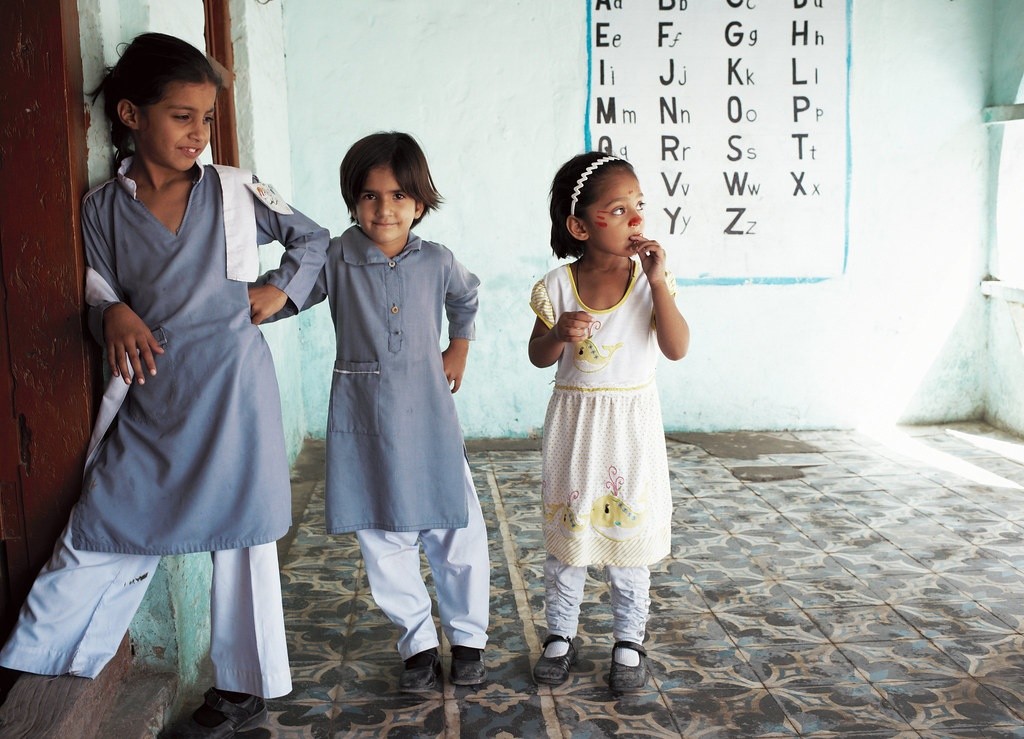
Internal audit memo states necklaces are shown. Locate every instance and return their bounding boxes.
[575,257,632,297]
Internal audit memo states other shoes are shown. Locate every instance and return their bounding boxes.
[166,687,267,739]
[533,633,578,684]
[449,644,488,686]
[609,641,647,692]
[399,648,440,693]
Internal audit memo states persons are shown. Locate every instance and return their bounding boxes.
[0,32,330,739]
[252,132,490,692]
[529,152,688,692]
